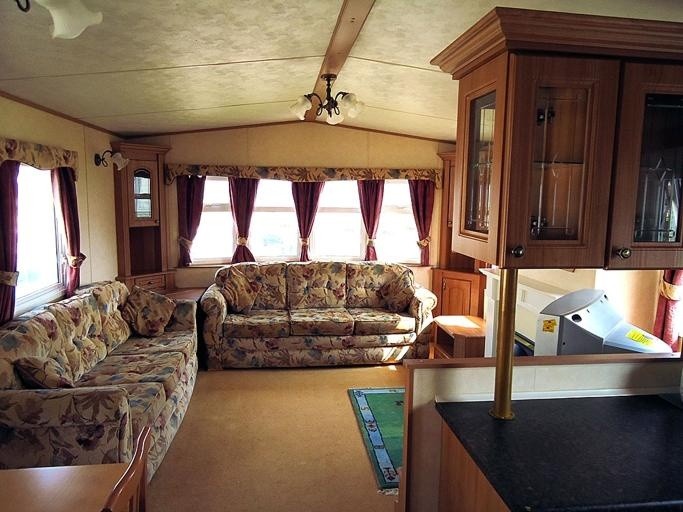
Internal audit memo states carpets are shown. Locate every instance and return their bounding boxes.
[347,386,405,490]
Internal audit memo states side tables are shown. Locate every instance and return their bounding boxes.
[431,314,485,359]
[164,287,209,331]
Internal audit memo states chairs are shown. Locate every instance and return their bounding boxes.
[102,425,152,511]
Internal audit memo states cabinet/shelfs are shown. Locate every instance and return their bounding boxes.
[110,141,177,294]
[429,48,681,345]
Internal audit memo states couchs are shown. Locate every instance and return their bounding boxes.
[0,261,197,485]
[200,260,438,371]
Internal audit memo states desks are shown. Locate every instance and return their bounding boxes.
[0,462,129,511]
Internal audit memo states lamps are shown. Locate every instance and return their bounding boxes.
[94,150,129,171]
[289,74,364,126]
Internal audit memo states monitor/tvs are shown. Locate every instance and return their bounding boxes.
[534,289,674,357]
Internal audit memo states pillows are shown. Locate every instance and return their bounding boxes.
[379,268,415,313]
[11,356,75,388]
[221,265,262,316]
[122,285,177,338]
